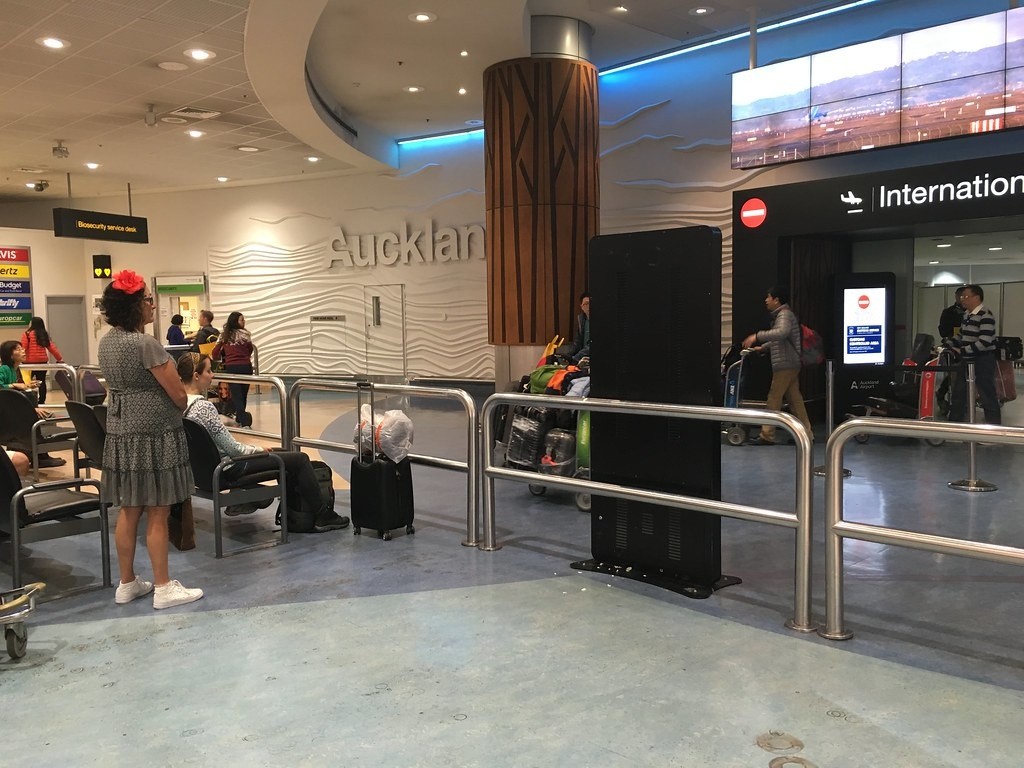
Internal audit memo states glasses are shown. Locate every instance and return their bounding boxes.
[959,295,975,299]
[142,297,153,305]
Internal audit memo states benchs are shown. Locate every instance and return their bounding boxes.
[0,389,288,612]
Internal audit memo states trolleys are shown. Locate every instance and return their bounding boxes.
[0,580,48,659]
[843,347,961,447]
[527,401,592,513]
[719,347,757,446]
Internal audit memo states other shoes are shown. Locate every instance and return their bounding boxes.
[31,456,66,468]
[749,436,774,445]
[224,498,274,516]
[935,391,944,408]
[37,399,45,404]
[788,438,815,445]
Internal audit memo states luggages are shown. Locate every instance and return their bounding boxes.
[54,360,107,406]
[350,382,415,541]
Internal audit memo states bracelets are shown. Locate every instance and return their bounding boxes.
[11,383,16,387]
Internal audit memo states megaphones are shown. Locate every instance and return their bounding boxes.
[34,183,49,192]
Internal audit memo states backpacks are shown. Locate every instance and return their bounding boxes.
[774,307,825,367]
[275,460,335,533]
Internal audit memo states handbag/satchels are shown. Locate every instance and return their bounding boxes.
[199,335,224,360]
[21,391,37,408]
[870,333,936,418]
[488,335,590,477]
[976,337,1022,403]
[721,341,773,409]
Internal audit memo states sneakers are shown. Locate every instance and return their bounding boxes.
[115,574,153,603]
[153,580,203,610]
[313,503,349,532]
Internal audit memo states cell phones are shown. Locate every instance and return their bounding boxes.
[28,381,42,387]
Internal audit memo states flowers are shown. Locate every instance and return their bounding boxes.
[109,267,145,298]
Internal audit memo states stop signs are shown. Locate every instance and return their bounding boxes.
[740,198,766,229]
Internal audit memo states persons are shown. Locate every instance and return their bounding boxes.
[217,381,231,401]
[569,292,589,360]
[935,287,967,404]
[177,352,350,531]
[742,288,814,447]
[167,314,193,345]
[6,451,29,476]
[97,270,203,609]
[20,317,63,404]
[937,284,1002,446]
[191,310,220,351]
[213,312,253,429]
[7,407,66,468]
[0,340,40,391]
